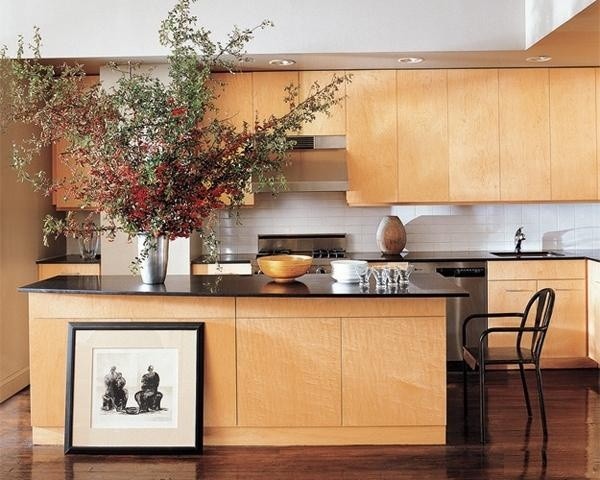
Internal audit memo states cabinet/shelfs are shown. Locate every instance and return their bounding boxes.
[489,259,586,360]
[49,74,103,213]
[34,253,101,281]
[237,316,447,430]
[585,261,600,366]
[30,318,239,429]
[192,73,254,208]
[252,70,345,137]
[344,67,600,206]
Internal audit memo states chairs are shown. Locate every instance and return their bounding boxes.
[461,288,555,445]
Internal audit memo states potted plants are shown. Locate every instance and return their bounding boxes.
[0,1,348,286]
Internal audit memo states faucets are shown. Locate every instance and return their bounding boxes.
[515,226,526,253]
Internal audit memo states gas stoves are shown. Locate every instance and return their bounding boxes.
[253,233,347,275]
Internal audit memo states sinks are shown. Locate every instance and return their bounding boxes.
[490,251,567,257]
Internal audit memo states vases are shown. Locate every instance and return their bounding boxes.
[78,221,98,260]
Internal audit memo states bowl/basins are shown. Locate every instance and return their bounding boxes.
[332,282,361,293]
[259,277,311,296]
[327,258,370,286]
[257,253,313,279]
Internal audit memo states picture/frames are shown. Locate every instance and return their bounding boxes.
[65,321,205,457]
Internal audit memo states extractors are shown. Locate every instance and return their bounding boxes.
[251,137,350,193]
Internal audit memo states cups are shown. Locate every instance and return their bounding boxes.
[371,265,387,288]
[397,264,416,285]
[385,267,399,287]
[355,264,370,286]
